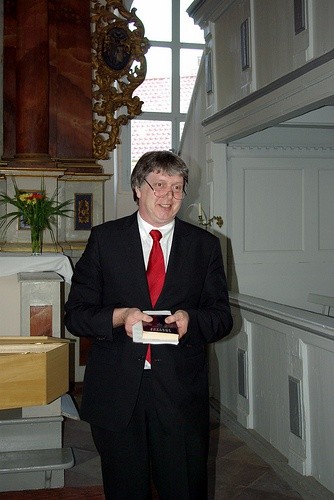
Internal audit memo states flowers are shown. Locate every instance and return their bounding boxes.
[0,172,76,256]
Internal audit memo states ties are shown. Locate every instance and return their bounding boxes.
[144,230,166,365]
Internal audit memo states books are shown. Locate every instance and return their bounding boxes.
[133,310,179,344]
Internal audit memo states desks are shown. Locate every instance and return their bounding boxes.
[0,251,73,286]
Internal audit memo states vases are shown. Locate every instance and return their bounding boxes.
[31,227,42,256]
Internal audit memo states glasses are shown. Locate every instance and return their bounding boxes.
[144,177,187,200]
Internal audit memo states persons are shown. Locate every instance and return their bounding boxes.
[63,150,234,500]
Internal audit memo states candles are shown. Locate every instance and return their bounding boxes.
[199,201,202,215]
